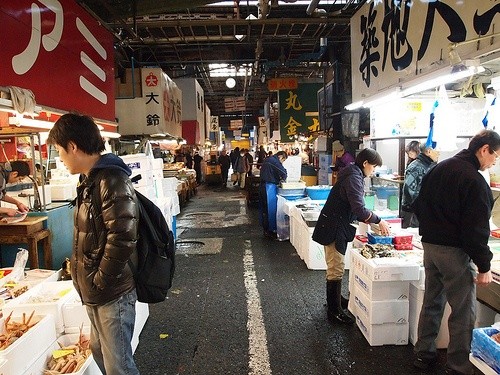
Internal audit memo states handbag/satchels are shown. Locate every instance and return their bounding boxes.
[231,172,237,181]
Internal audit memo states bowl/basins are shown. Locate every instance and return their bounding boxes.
[306,189,332,200]
[281,195,306,201]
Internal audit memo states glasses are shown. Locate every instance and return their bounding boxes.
[19,176,23,183]
[481,142,500,160]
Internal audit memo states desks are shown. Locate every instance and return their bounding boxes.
[0,229,52,271]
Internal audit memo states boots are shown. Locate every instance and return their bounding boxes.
[341,295,349,310]
[327,280,356,324]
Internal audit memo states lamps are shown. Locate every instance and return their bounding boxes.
[400,58,486,100]
[448,32,500,77]
[319,37,327,46]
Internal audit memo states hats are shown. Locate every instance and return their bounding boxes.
[332,140,343,152]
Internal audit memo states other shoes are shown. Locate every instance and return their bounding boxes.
[441,362,485,375]
[269,233,277,239]
[263,232,269,238]
[414,350,440,368]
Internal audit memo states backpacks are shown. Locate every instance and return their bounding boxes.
[93,167,175,303]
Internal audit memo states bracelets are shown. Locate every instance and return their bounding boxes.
[17,202,23,207]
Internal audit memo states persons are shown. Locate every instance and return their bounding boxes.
[46,113,142,375]
[35,164,45,185]
[414,130,500,375]
[400,140,439,227]
[333,140,355,184]
[311,147,391,324]
[260,150,289,237]
[173,146,274,188]
[0,160,31,217]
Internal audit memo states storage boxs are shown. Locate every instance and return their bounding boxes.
[318,154,332,185]
[469,326,500,375]
[346,235,452,349]
[0,279,150,375]
[114,67,141,97]
